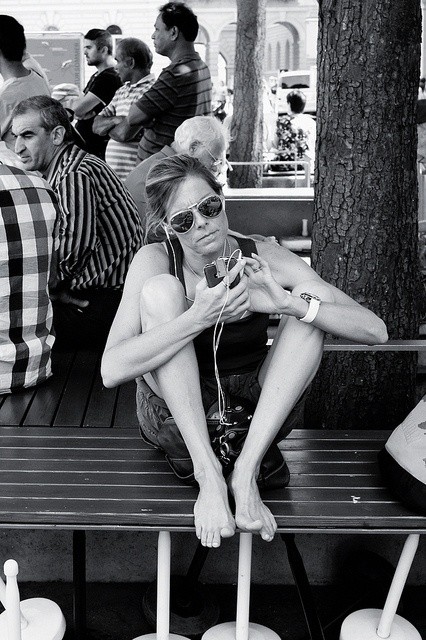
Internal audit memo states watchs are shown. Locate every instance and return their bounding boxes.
[296,293,321,323]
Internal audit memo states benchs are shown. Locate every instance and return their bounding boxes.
[0,426,426,640]
[2,329,138,430]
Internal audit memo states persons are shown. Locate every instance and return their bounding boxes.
[92,38,158,182]
[11,96,145,358]
[51,83,80,103]
[0,15,52,152]
[266,91,316,172]
[0,162,61,394]
[127,2,211,165]
[125,115,233,244]
[69,29,124,161]
[102,153,388,547]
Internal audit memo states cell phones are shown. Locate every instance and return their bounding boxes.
[206,258,241,296]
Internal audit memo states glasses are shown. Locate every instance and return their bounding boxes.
[159,191,224,236]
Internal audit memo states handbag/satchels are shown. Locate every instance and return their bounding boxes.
[154,380,292,493]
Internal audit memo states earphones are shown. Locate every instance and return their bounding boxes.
[163,223,243,428]
